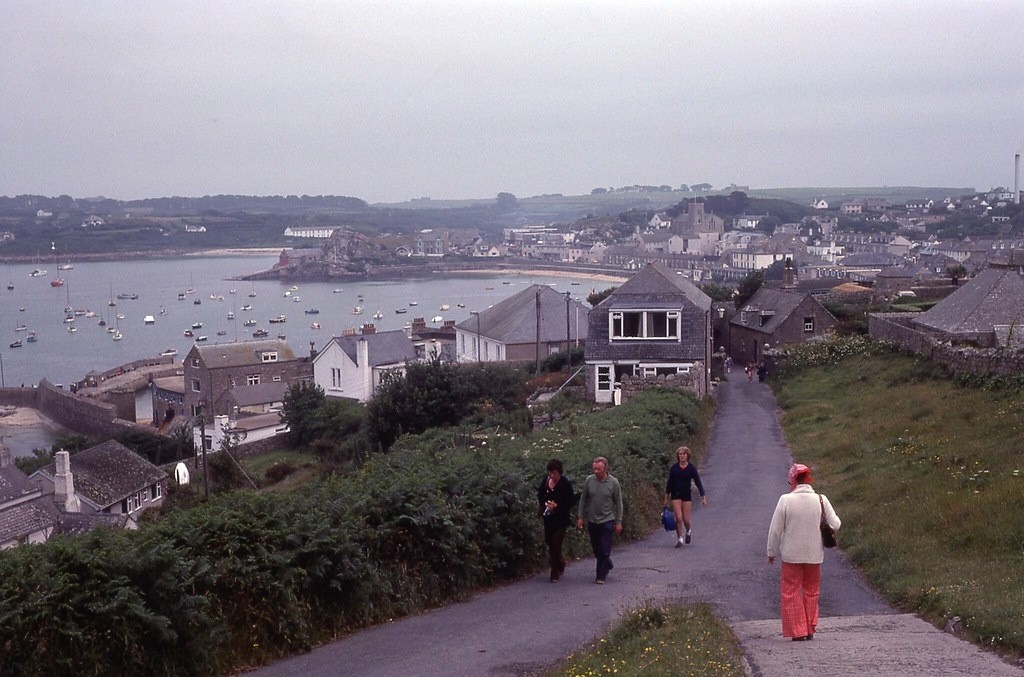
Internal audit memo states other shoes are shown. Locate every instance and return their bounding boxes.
[551,558,566,583]
[792,634,813,641]
[675,542,682,548]
[596,561,613,583]
[685,533,691,544]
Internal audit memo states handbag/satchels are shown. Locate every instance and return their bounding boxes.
[818,493,837,548]
[661,506,676,532]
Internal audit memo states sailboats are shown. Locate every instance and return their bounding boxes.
[484,280,493,290]
[8,242,126,347]
[186,271,196,294]
[456,289,465,308]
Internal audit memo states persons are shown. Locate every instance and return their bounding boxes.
[578,457,626,585]
[766,462,842,642]
[537,460,578,582]
[745,359,769,384]
[660,444,709,547]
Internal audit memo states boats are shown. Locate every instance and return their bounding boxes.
[522,280,535,284]
[161,349,178,356]
[144,315,156,324]
[185,285,450,344]
[503,282,511,285]
[543,283,557,286]
[572,281,579,285]
[160,309,167,315]
[119,293,139,299]
[177,293,186,300]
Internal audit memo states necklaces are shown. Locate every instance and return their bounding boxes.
[680,462,687,465]
